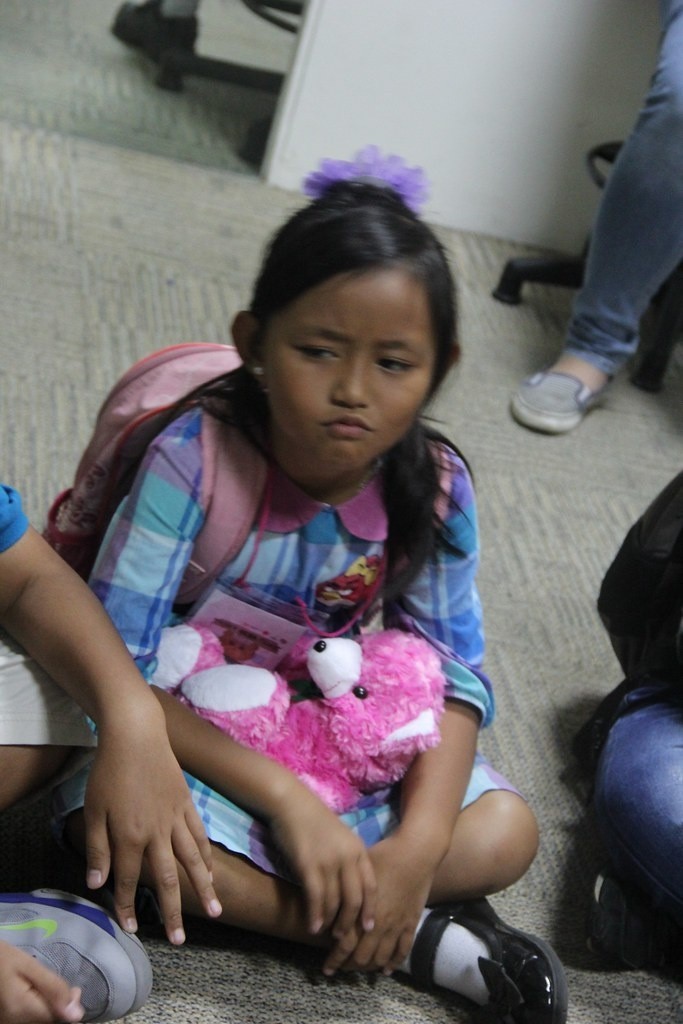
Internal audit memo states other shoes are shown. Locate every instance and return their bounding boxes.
[512,369,607,433]
[584,868,682,983]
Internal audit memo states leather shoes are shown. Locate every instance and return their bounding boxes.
[377,898,567,1023]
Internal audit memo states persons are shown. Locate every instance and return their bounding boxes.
[570,469,683,969]
[0,477,222,1024]
[92,154,567,1024]
[511,0,683,433]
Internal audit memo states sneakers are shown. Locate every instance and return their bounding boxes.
[1,888,154,1023]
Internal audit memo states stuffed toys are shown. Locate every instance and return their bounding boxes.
[152,618,445,814]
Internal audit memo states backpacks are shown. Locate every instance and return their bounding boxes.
[42,341,450,618]
